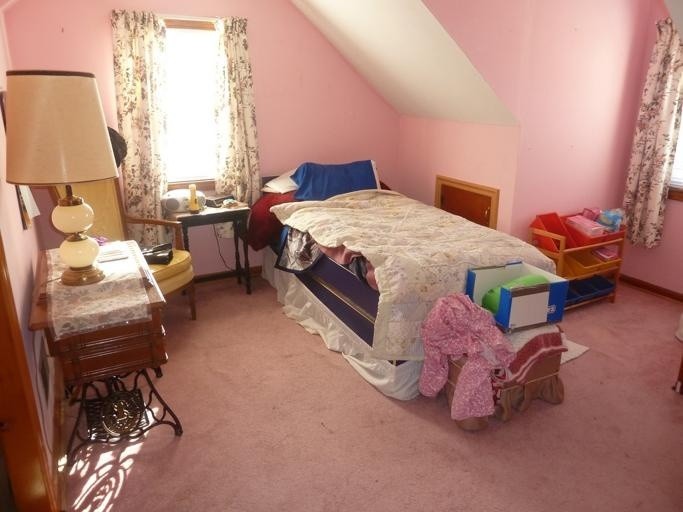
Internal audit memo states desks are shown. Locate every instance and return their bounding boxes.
[172,201,251,296]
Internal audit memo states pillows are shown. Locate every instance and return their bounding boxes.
[237,190,303,251]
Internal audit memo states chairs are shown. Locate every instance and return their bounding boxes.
[48,179,197,320]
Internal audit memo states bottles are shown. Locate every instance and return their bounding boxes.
[188,184,199,214]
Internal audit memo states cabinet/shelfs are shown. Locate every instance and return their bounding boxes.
[27,240,183,466]
[528,224,627,312]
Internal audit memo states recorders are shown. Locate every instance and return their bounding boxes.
[159,188,206,213]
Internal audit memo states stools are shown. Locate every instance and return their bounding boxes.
[443,321,568,431]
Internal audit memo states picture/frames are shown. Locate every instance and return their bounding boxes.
[434,175,498,230]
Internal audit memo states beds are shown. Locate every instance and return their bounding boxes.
[260,176,557,401]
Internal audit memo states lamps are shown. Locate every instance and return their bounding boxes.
[6,70,120,286]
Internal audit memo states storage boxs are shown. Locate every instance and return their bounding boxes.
[466,263,569,334]
